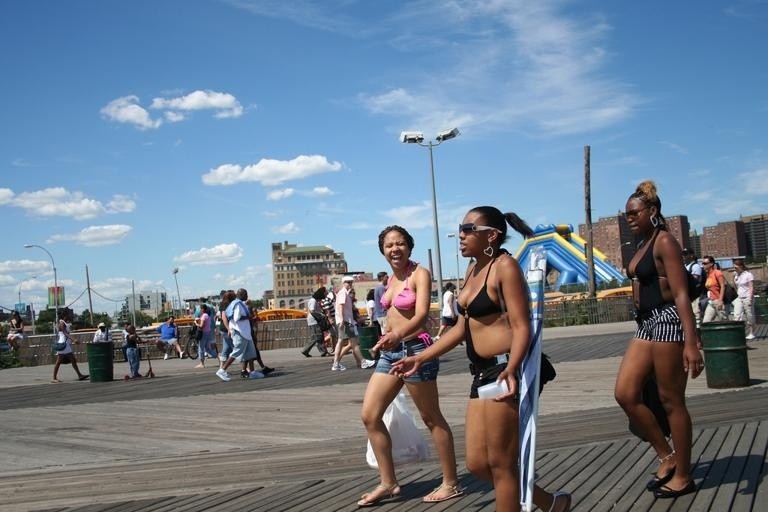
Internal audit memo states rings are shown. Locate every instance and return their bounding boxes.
[699,363,704,367]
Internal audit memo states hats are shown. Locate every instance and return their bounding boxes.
[342,276,354,284]
[98,323,105,328]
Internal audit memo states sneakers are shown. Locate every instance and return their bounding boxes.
[241,371,264,378]
[216,370,231,381]
[361,359,375,368]
[332,364,346,371]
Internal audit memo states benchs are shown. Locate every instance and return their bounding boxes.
[104,327,180,360]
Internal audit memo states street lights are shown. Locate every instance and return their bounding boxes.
[447,231,462,296]
[173,267,184,316]
[400,126,455,308]
[24,243,59,334]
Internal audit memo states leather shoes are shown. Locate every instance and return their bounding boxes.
[647,465,696,498]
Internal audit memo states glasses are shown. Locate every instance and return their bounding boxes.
[459,224,503,234]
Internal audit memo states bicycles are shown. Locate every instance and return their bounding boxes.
[187,334,220,360]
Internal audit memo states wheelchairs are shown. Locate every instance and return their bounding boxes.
[317,322,338,357]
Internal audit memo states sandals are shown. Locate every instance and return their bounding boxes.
[549,491,571,511]
[358,483,403,506]
[423,483,463,502]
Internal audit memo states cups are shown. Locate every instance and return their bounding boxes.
[477,379,510,400]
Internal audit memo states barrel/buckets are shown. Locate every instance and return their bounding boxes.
[701,319,750,389]
[85,341,115,383]
[357,326,381,363]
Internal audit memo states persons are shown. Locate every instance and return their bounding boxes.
[433,282,463,345]
[730,259,757,340]
[614,181,705,500]
[357,226,464,507]
[7,311,24,352]
[681,247,703,350]
[389,206,571,512]
[50,308,89,383]
[93,322,112,343]
[702,255,729,324]
[120,271,387,382]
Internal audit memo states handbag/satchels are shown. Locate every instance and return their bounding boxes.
[722,284,738,305]
[318,317,331,331]
[196,329,203,340]
[53,343,66,351]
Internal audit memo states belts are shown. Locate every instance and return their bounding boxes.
[469,349,509,375]
[399,338,424,350]
